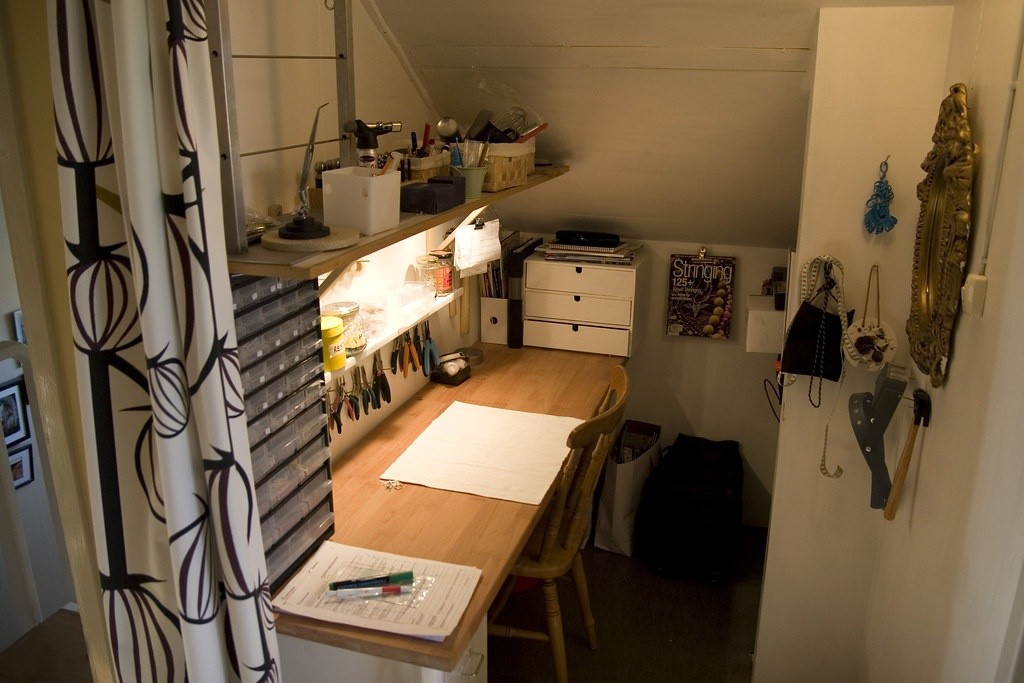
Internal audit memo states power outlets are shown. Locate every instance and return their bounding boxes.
[960,274,987,317]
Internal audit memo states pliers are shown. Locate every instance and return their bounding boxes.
[391,319,440,379]
[328,348,392,442]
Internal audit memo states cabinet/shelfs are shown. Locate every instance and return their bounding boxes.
[229,273,335,596]
[523,252,652,358]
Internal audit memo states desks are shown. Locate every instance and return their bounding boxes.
[269,339,629,683]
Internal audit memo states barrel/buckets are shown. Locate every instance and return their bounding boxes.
[449,165,487,199]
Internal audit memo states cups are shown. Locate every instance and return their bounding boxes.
[412,255,439,307]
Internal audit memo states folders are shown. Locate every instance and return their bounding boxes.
[507,236,544,349]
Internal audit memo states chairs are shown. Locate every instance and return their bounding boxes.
[487,365,630,683]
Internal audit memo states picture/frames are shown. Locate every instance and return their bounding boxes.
[0,376,31,449]
[8,443,35,490]
[12,309,27,367]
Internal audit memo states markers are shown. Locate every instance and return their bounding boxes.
[328,570,414,591]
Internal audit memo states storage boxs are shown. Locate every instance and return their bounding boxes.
[449,143,527,192]
[408,151,451,182]
[480,296,508,345]
[322,166,401,236]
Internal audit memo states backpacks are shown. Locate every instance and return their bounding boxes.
[635,432,742,584]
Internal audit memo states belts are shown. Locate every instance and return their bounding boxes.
[849,392,891,509]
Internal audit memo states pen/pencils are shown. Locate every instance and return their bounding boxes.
[455,137,464,167]
[337,586,416,597]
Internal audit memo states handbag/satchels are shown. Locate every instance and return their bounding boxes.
[780,259,855,382]
[594,419,661,557]
[842,265,897,373]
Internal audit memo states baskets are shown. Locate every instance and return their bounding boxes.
[395,131,536,193]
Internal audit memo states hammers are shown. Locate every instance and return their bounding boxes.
[444,227,470,335]
[882,388,932,522]
